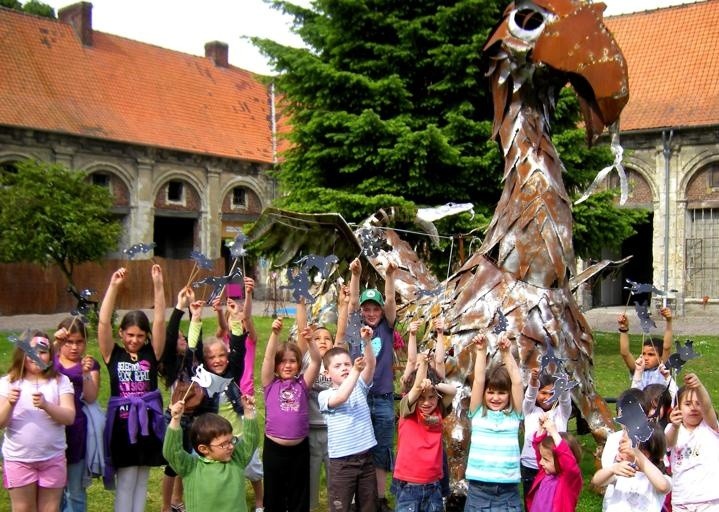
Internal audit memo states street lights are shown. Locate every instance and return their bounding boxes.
[662,129,682,312]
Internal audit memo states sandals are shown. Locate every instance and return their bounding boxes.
[170,502,186,512]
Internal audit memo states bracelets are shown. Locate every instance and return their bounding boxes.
[619,328,628,333]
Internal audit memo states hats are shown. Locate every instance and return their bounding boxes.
[359,288,384,307]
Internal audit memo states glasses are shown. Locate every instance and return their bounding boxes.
[63,341,84,347]
[206,435,238,448]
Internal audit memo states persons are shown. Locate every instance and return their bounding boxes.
[600,388,651,469]
[393,353,457,512]
[591,422,672,512]
[0,329,75,512]
[218,277,264,512]
[290,267,351,512]
[159,380,205,512]
[262,319,321,512]
[317,322,379,512]
[464,334,524,512]
[618,309,679,412]
[98,261,165,512]
[523,368,573,511]
[526,414,585,512]
[666,373,719,512]
[49,316,102,512]
[160,288,195,512]
[163,396,259,512]
[391,318,451,512]
[185,299,263,512]
[642,385,675,512]
[348,257,397,512]
